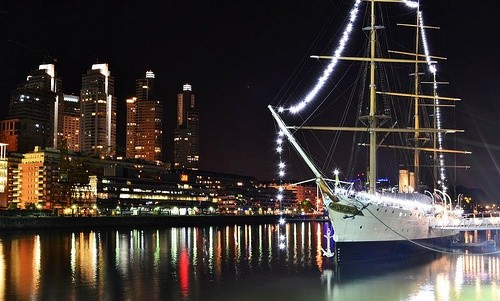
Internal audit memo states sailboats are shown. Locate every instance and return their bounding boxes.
[320,240,461,300]
[267,0,499,275]
